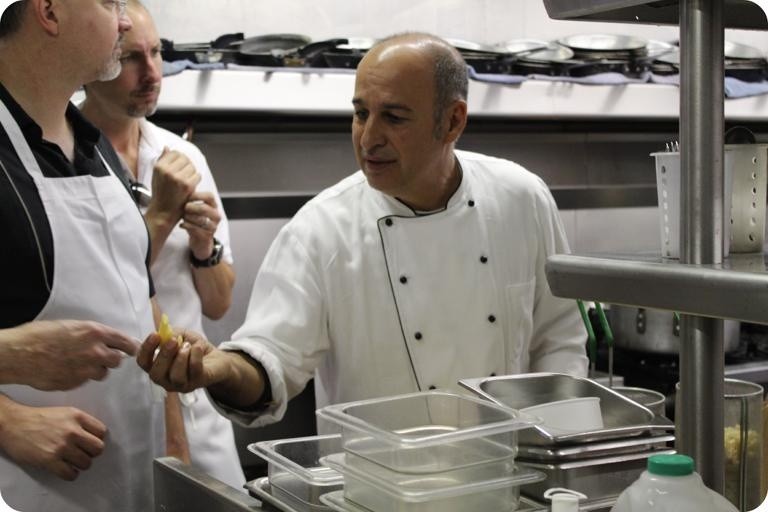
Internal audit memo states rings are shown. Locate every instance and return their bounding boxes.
[202,216,208,228]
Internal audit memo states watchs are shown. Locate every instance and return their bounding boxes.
[189,237,224,267]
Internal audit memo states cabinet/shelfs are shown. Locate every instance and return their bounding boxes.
[542,0,767,512]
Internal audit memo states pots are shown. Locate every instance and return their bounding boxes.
[446,30,765,79]
[160,30,349,68]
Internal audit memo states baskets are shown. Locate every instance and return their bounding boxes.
[649,140,768,261]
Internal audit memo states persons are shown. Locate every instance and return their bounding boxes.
[73,0,249,499]
[133,29,592,467]
[0,0,191,512]
[0,321,144,393]
